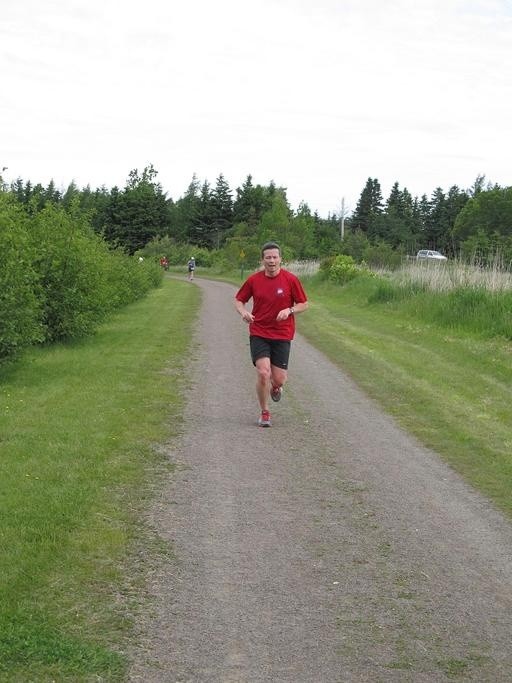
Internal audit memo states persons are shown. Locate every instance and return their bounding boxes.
[160,256,169,270]
[234,242,309,428]
[186,256,196,280]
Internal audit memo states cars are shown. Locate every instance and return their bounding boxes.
[418,249,448,261]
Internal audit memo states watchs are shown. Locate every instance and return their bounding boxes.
[288,307,294,315]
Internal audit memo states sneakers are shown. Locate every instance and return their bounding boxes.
[270,381,282,402]
[258,410,271,427]
